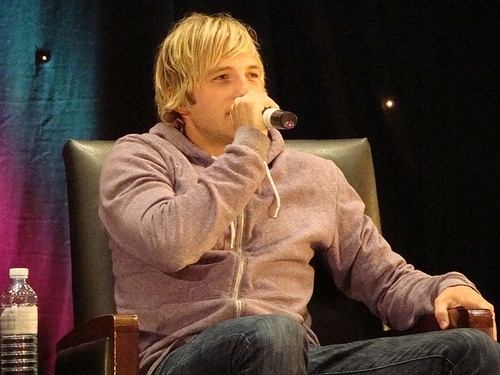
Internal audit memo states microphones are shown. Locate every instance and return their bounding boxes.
[262,106,298,129]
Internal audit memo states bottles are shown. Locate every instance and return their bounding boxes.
[0,267,39,375]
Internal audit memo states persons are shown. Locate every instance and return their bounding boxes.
[98,12,500,375]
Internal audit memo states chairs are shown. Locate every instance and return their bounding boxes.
[54,137,493,375]
